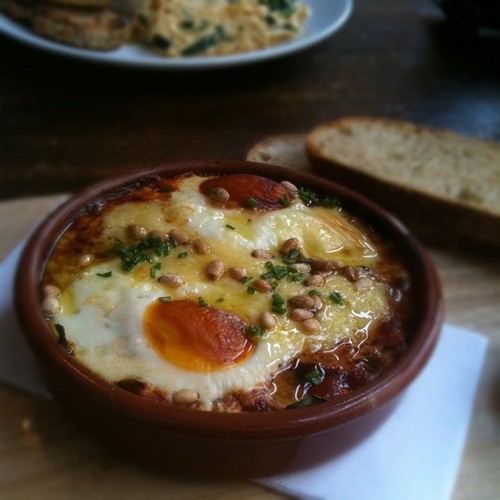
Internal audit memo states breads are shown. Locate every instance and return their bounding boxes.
[244,114,499,250]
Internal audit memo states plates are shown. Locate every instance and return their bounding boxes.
[2,0,359,69]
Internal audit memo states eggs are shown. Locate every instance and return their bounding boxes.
[43,175,393,405]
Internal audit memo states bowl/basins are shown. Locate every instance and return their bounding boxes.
[14,156,446,483]
